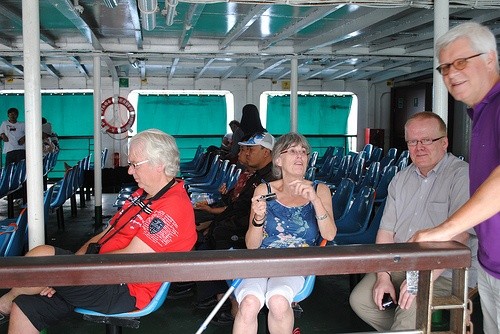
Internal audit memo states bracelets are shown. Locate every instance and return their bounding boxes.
[252,219,265,227]
[386,272,393,281]
[315,211,329,220]
[253,213,266,221]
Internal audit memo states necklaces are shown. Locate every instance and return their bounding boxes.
[86,195,154,255]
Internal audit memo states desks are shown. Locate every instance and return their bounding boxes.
[84,166,138,195]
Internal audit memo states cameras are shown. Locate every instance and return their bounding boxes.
[85,243,100,254]
[382,293,394,308]
[258,192,277,202]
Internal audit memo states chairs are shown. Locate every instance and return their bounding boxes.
[74,281,172,334]
[0,144,465,256]
[224,237,327,334]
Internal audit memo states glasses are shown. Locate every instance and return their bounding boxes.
[406,136,444,145]
[436,52,485,76]
[279,149,307,156]
[128,159,150,169]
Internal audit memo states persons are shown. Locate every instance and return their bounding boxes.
[0,107,25,166]
[232,133,337,334]
[42,117,59,189]
[407,21,500,334]
[227,104,268,164]
[221,120,240,153]
[350,112,479,334]
[190,133,276,325]
[166,135,256,300]
[0,129,197,334]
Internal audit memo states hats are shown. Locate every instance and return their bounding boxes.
[238,131,277,152]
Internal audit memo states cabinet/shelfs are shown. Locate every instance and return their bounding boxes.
[364,128,385,158]
